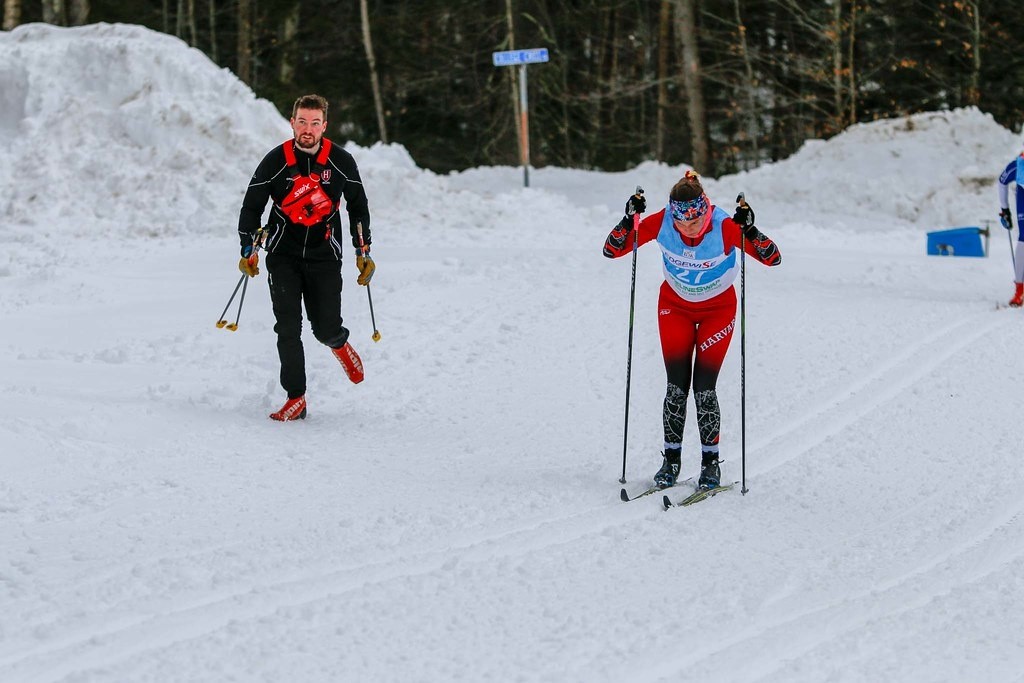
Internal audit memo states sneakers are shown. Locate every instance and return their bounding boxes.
[331,341,365,385]
[1009,282,1024,307]
[653,446,682,489]
[697,451,725,492]
[268,395,307,422]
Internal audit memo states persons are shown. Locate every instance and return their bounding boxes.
[238,95,377,421]
[997,152,1024,308]
[602,170,781,488]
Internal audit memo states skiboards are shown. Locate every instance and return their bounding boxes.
[994,301,1018,310]
[619,475,742,509]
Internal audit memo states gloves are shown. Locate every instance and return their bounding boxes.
[732,202,758,241]
[354,244,376,286]
[623,193,646,223]
[999,207,1013,230]
[238,249,259,278]
